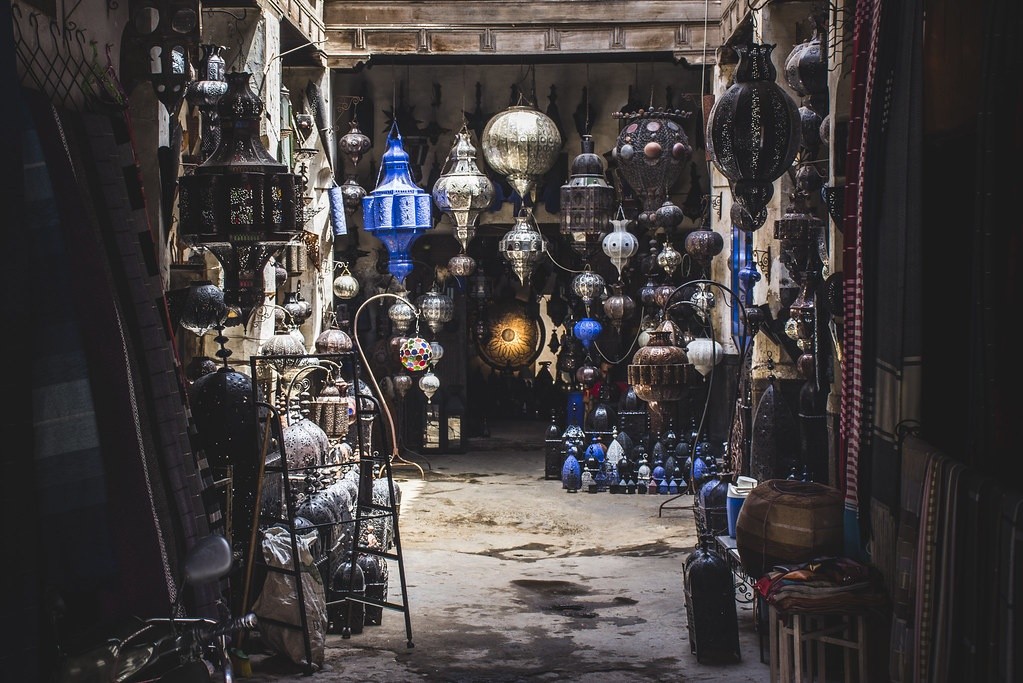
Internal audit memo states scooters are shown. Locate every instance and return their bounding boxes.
[55,539,260,683]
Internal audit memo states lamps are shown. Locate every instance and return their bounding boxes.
[144,0,829,664]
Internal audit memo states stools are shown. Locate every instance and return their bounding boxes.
[753,555,875,683]
